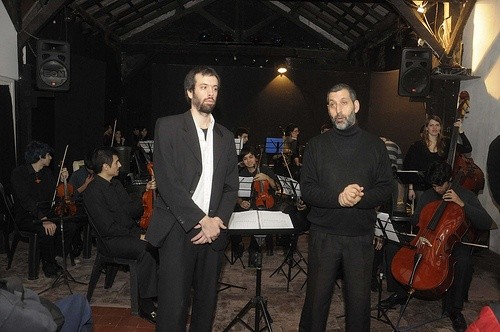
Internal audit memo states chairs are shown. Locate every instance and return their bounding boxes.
[2,186,76,280]
[83,202,140,317]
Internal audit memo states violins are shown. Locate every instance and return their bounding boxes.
[55,168,77,217]
[250,164,274,209]
[139,164,157,230]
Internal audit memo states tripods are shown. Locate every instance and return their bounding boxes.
[269,207,310,292]
[335,215,401,332]
[47,202,83,294]
[221,228,297,331]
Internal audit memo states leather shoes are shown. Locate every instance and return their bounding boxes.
[380,293,409,305]
[447,308,467,332]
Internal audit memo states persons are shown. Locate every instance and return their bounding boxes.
[145,67,239,332]
[0,276,93,332]
[403,115,472,224]
[10,141,94,278]
[486,135,500,212]
[298,83,393,332]
[234,123,335,171]
[82,147,158,322]
[232,146,308,257]
[104,125,150,171]
[379,123,429,178]
[376,163,492,332]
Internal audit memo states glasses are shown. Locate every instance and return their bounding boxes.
[326,100,353,109]
[292,130,300,133]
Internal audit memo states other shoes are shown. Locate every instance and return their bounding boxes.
[74,240,84,257]
[42,255,63,278]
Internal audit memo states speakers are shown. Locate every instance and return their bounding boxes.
[33,40,71,90]
[399,47,433,96]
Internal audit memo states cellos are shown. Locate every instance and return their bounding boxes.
[392,156,472,330]
[446,91,485,197]
